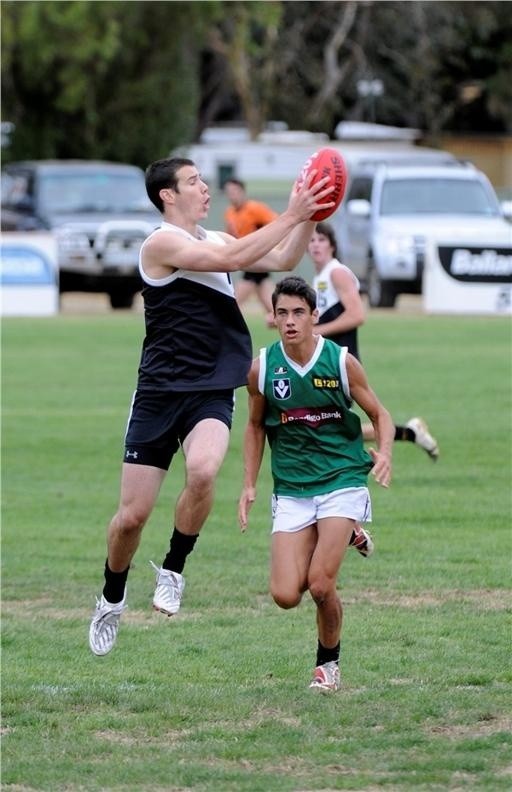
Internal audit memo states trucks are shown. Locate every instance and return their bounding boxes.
[168,117,426,300]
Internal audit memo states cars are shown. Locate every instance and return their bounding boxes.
[2,195,51,234]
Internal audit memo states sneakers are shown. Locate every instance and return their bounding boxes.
[89,583,127,656]
[309,660,341,694]
[149,560,184,615]
[406,417,439,457]
[348,521,374,557]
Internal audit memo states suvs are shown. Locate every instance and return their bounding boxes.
[1,154,167,310]
[329,145,512,310]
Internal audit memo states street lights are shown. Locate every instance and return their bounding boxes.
[354,75,388,123]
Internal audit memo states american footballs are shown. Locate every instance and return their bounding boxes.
[296,148,347,222]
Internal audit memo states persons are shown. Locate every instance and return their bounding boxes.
[238,276,396,696]
[89,157,336,657]
[266,220,440,462]
[221,178,280,329]
